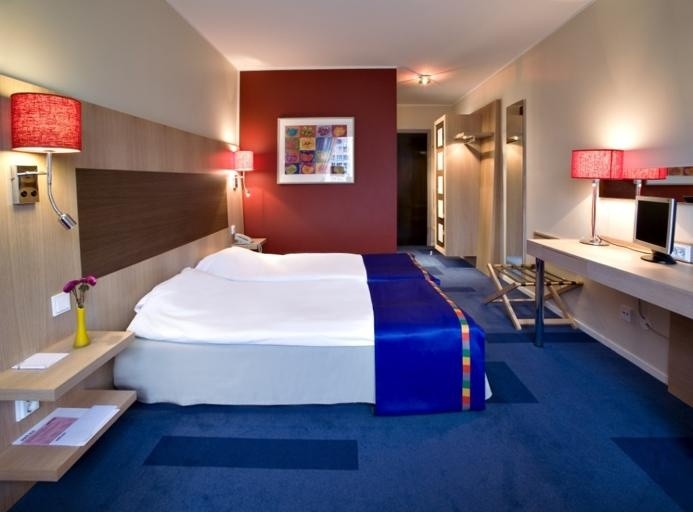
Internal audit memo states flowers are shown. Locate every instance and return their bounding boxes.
[63,276,97,308]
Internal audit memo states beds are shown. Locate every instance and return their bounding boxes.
[194,246,441,283]
[112,265,493,414]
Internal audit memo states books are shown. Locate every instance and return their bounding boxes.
[7,350,68,370]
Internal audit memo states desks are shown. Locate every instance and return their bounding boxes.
[526,236,693,347]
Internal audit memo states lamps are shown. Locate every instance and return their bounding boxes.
[228,151,254,197]
[10,94,82,230]
[418,76,431,86]
[571,149,624,246]
[622,168,667,200]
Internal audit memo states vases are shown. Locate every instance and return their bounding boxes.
[73,305,91,349]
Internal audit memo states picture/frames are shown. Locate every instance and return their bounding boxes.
[277,116,354,184]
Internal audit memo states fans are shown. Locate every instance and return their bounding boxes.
[398,66,456,89]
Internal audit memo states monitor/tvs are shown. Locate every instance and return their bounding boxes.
[633,195,677,265]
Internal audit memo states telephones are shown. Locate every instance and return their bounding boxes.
[234,233,251,245]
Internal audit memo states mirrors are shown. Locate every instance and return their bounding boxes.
[598,166,693,203]
[505,98,526,278]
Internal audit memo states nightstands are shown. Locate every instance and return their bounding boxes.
[231,237,267,252]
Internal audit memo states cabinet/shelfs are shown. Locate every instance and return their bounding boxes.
[0,328,138,482]
[430,113,479,258]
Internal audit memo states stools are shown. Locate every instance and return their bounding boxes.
[479,262,583,333]
